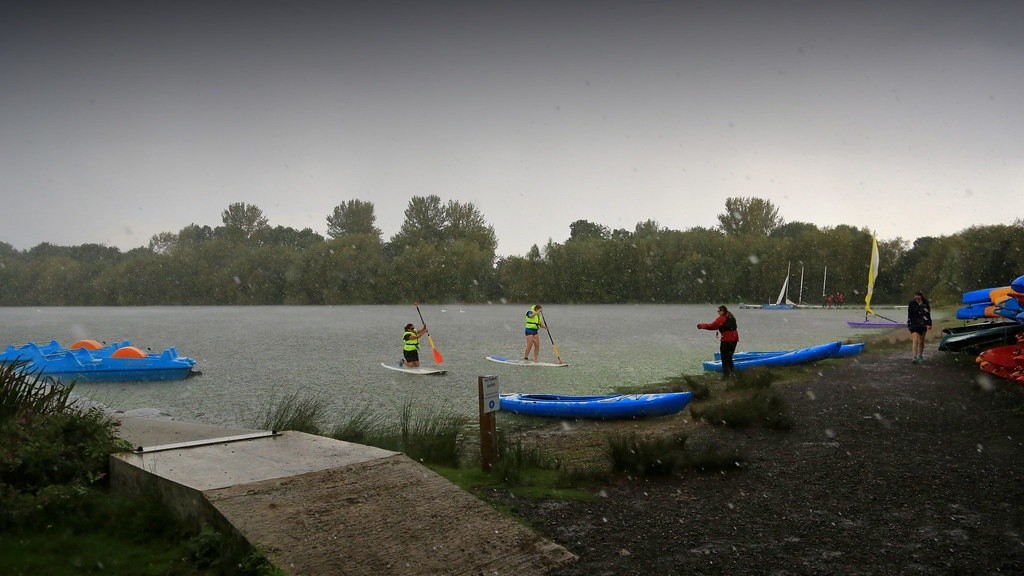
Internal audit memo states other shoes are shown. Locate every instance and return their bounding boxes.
[918,356,924,362]
[718,374,729,380]
[399,359,404,367]
[912,356,917,363]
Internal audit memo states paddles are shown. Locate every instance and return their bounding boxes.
[541,311,563,365]
[415,304,443,364]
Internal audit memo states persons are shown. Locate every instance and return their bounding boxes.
[697,305,739,381]
[907,291,932,364]
[398,323,428,367]
[821,292,843,307]
[524,305,547,364]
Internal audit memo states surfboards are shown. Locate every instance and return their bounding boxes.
[485,356,571,367]
[382,363,448,375]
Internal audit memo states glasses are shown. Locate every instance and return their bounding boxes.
[718,310,722,313]
[407,328,414,330]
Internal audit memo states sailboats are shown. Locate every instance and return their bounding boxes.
[847,230,909,328]
[762,276,794,310]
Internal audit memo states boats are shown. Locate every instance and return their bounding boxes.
[957,275,1024,326]
[714,342,864,358]
[0,340,131,363]
[498,391,691,419]
[16,347,196,381]
[703,341,842,372]
